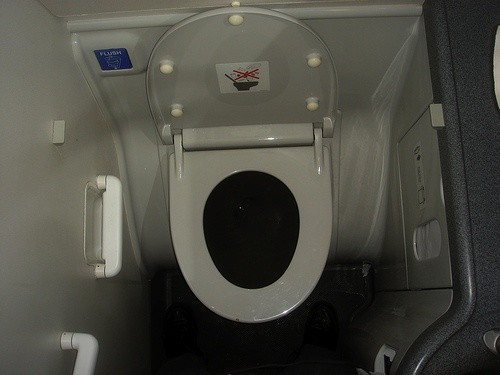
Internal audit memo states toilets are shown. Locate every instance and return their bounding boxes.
[144,7,338,323]
